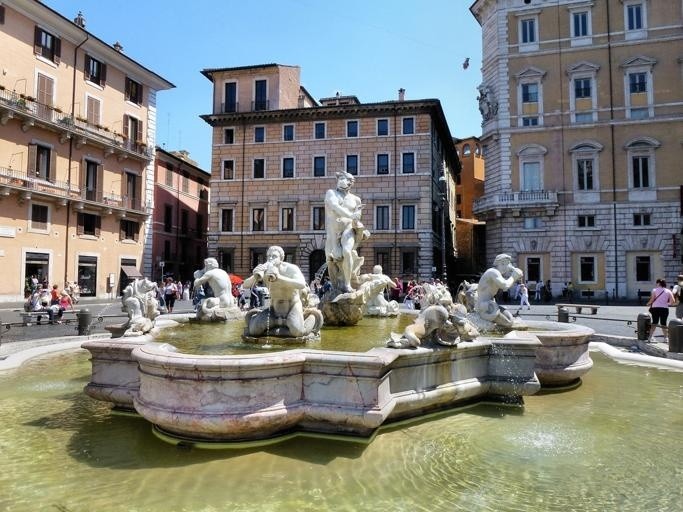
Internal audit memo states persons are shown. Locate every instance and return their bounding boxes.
[533,278,552,303]
[243,246,315,337]
[318,279,332,300]
[233,282,261,311]
[646,278,674,343]
[464,254,522,329]
[193,257,234,314]
[24,274,78,325]
[672,281,681,304]
[563,282,573,302]
[457,279,475,305]
[676,275,683,322]
[358,265,397,317]
[514,277,530,310]
[324,170,371,301]
[383,278,448,304]
[158,277,204,312]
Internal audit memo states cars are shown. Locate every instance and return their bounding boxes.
[238,280,270,299]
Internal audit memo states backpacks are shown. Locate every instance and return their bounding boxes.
[23,301,32,312]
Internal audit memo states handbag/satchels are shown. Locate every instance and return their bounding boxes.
[649,307,655,314]
[173,291,179,299]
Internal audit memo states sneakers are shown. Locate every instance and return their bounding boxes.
[665,338,669,344]
[649,337,658,344]
[36,319,63,325]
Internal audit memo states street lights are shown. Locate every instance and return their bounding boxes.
[437,174,450,284]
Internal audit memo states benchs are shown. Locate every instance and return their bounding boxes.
[18,308,83,324]
[554,303,601,315]
